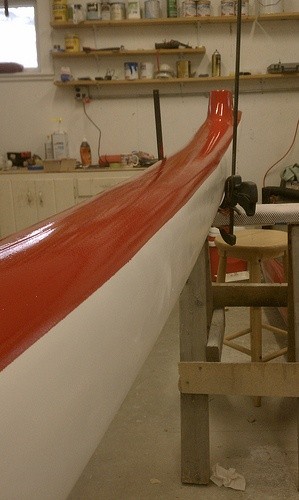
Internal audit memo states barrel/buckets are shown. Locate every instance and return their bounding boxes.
[124,62,139,80]
[139,63,154,79]
[183,0,197,17]
[64,32,80,53]
[255,0,284,14]
[50,0,67,23]
[61,65,72,81]
[221,0,234,16]
[197,0,210,17]
[144,0,160,19]
[87,3,98,19]
[234,0,249,16]
[110,2,125,20]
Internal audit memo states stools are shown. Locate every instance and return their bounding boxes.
[215,229,289,408]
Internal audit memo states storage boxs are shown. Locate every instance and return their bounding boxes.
[44,158,76,170]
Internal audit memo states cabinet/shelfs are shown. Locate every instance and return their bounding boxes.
[53,173,74,214]
[74,171,144,204]
[11,173,56,232]
[50,11,299,85]
[0,174,15,241]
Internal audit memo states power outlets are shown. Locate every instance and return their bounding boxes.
[75,87,88,100]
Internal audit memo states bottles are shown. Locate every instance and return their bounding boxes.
[80,138,92,169]
[212,49,221,77]
